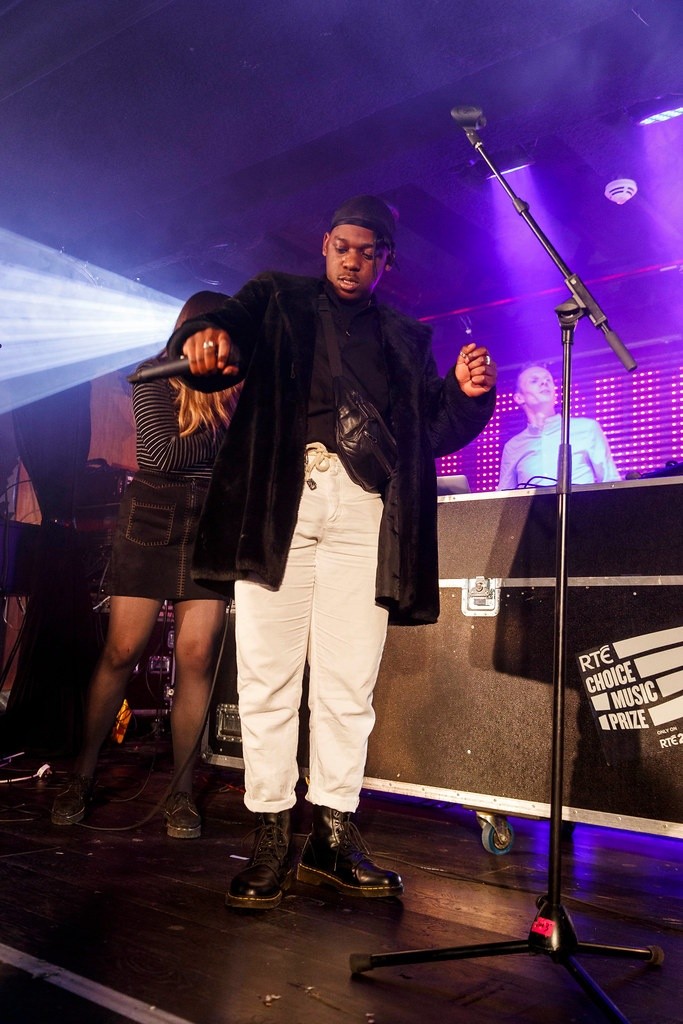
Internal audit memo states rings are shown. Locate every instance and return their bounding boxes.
[203,340,217,349]
[460,351,466,358]
[486,355,491,366]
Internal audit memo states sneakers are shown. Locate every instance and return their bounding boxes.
[164,791,202,838]
[51,776,99,826]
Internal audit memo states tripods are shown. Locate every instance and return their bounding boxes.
[348,105,664,1024]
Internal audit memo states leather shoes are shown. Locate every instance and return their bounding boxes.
[224,811,294,909]
[298,804,404,897]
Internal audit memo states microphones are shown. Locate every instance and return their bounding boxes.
[127,346,240,384]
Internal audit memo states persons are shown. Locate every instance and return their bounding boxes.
[51,290,250,839]
[496,360,622,491]
[158,200,494,912]
